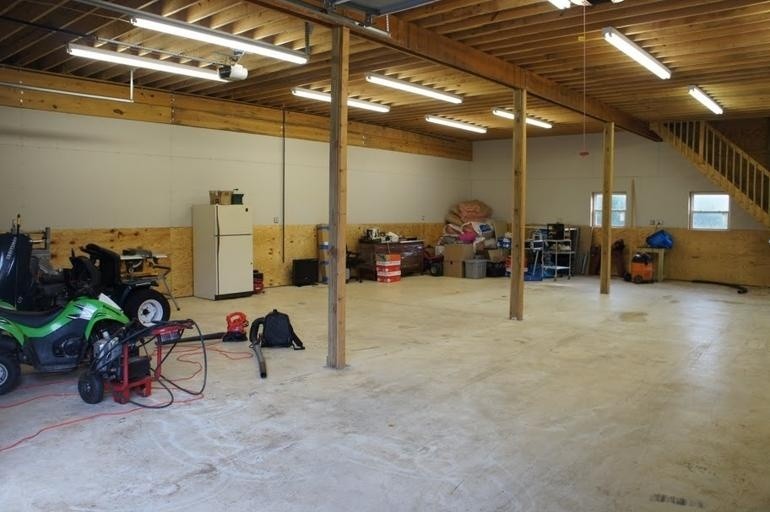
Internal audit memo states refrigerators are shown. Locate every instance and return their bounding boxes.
[190,204,254,300]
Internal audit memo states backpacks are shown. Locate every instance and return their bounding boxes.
[251,309,305,351]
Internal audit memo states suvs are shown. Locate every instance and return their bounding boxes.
[0,209,171,330]
[0,293,131,394]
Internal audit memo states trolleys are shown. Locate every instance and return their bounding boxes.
[78,320,198,404]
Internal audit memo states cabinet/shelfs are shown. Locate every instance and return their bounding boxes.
[524,225,580,280]
[358,241,425,282]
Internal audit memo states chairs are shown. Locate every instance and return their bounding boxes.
[344,244,364,284]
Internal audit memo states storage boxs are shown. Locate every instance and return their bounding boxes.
[444,244,474,262]
[464,259,490,278]
[442,260,465,277]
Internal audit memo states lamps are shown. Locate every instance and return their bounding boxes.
[365,72,464,105]
[688,84,724,116]
[67,42,230,85]
[129,15,310,67]
[548,1,592,11]
[290,86,390,113]
[491,107,554,129]
[424,114,488,134]
[598,24,673,80]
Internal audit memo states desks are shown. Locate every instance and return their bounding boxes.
[629,245,667,284]
